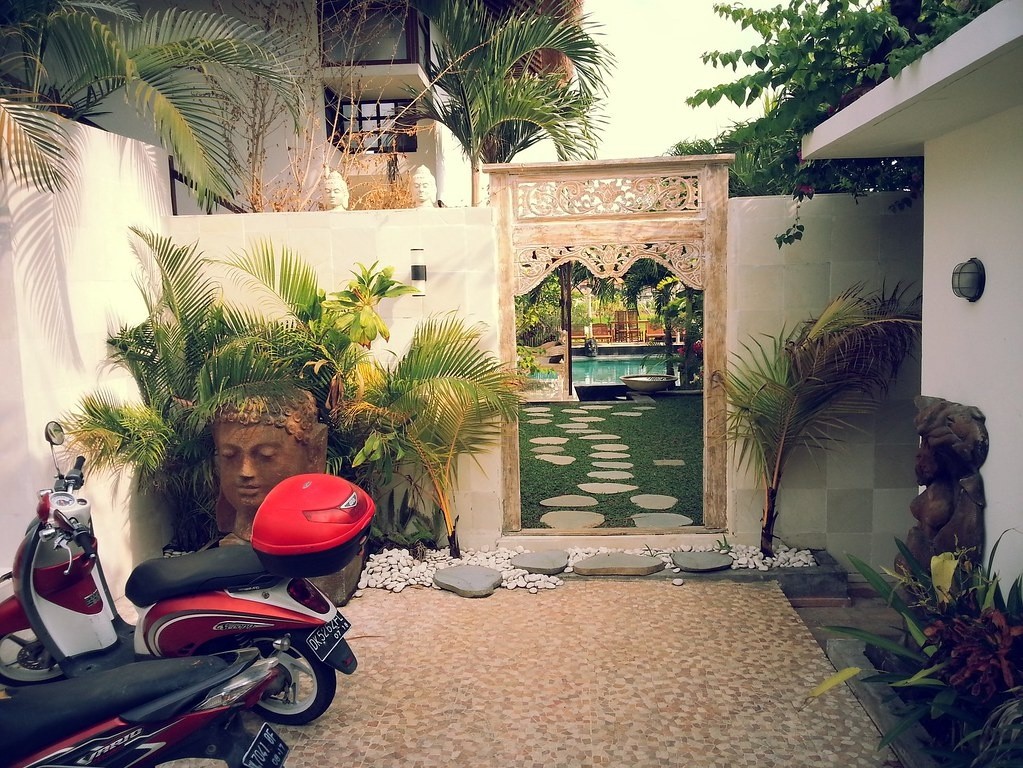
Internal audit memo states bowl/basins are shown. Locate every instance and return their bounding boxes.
[619,374,678,392]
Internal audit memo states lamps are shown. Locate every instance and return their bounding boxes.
[410,246,428,299]
[951,255,987,303]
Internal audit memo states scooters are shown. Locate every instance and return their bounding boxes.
[0,421,378,725]
[0,631,288,768]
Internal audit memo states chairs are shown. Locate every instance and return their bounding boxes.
[560,310,688,345]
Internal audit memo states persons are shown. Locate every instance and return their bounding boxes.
[888,392,988,654]
[324,170,350,210]
[410,164,437,207]
[206,385,329,542]
